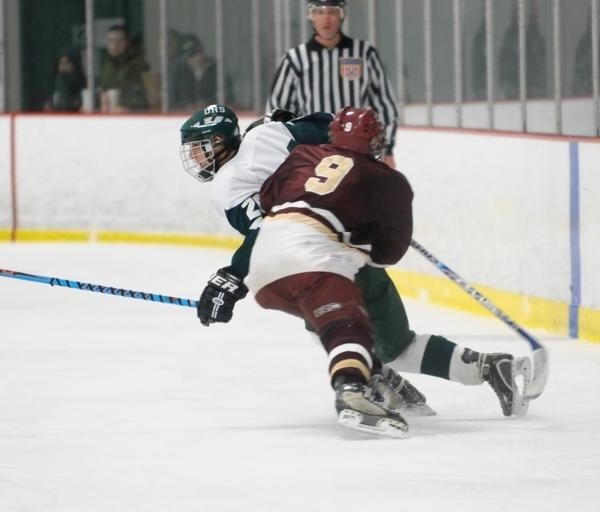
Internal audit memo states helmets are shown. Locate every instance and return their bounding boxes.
[307,0,346,23]
[180,104,240,182]
[327,105,387,162]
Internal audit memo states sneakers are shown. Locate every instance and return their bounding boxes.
[335,381,409,435]
[479,351,514,417]
[371,368,427,407]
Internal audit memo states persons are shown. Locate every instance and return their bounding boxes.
[262,0,401,171]
[36,21,233,112]
[248,104,436,437]
[179,104,550,419]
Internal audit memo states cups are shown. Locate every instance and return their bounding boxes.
[105,89,120,113]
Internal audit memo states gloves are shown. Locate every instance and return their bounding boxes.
[197,268,250,327]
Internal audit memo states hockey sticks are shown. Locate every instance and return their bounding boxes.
[409,239,540,350]
